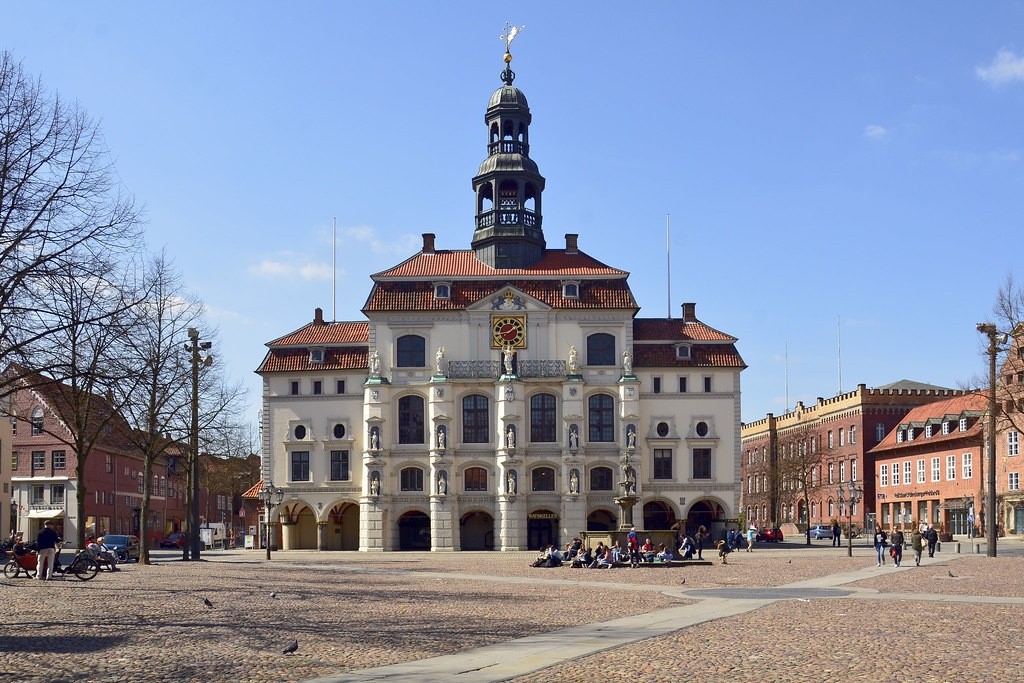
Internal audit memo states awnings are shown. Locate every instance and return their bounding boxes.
[25,509,65,519]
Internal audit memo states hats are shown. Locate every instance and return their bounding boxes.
[929,524,933,528]
[631,527,636,532]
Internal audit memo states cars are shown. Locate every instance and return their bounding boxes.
[99,535,140,564]
[159,533,192,549]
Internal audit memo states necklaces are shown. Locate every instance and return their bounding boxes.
[83,536,94,546]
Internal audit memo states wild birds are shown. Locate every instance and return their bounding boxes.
[270,590,277,599]
[948,570,953,577]
[787,560,792,563]
[204,598,213,609]
[680,579,686,584]
[283,639,299,655]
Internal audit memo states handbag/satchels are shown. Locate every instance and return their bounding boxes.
[892,546,896,558]
[627,542,633,548]
[921,535,929,547]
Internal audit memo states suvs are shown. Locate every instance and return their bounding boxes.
[756,528,783,541]
[806,525,833,539]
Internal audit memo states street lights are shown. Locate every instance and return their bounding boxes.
[259,479,284,559]
[837,480,864,556]
[962,493,975,537]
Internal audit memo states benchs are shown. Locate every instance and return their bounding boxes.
[85,545,111,570]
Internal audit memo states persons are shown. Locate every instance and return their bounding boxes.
[891,525,904,568]
[86,535,121,572]
[873,522,887,567]
[372,431,378,448]
[436,347,445,371]
[623,351,632,375]
[832,519,841,547]
[374,351,379,369]
[911,520,937,566]
[508,474,515,494]
[529,522,709,569]
[717,526,757,565]
[570,430,578,447]
[10,520,60,581]
[506,428,514,445]
[438,430,444,447]
[438,475,446,493]
[502,346,513,375]
[627,428,636,447]
[571,471,578,492]
[569,346,578,369]
[371,477,379,496]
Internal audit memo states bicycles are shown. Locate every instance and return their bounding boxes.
[53,540,100,581]
[0,529,33,579]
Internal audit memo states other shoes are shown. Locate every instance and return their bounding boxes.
[582,564,584,568]
[608,564,612,569]
[631,564,635,568]
[877,563,880,567]
[894,564,897,567]
[642,558,644,562]
[883,561,885,565]
[636,565,640,568]
[585,564,587,568]
[112,568,120,571]
[898,561,900,566]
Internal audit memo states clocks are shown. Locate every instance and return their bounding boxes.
[490,314,527,349]
